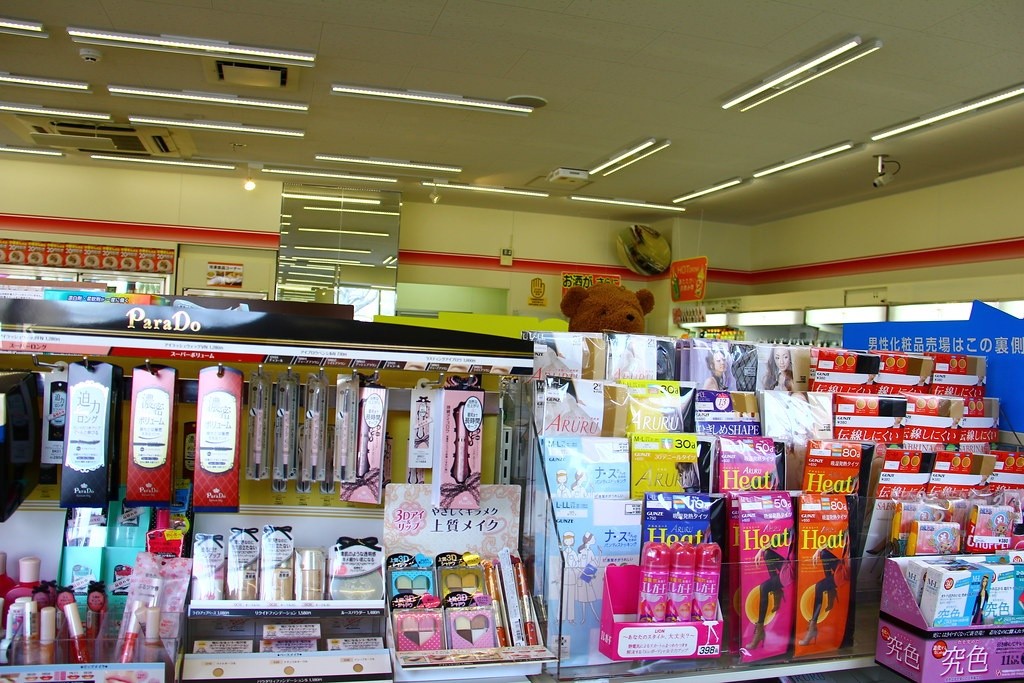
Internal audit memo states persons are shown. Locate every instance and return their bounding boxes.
[764,345,793,391]
[798,523,850,646]
[745,524,795,650]
[703,349,727,390]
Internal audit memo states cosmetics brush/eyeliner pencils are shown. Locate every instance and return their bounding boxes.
[486,548,538,646]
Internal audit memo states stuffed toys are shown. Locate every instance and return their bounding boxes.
[560,283,655,334]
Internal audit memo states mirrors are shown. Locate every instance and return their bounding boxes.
[274,181,400,323]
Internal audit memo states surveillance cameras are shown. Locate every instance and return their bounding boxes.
[873,173,894,187]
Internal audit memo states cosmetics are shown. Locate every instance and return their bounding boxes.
[190,526,496,654]
[0,552,57,629]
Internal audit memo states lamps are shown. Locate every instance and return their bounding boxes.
[588,137,656,176]
[129,115,305,138]
[0,18,50,38]
[90,155,236,169]
[0,145,62,156]
[720,36,863,110]
[314,153,462,173]
[870,82,1024,141]
[422,181,549,198]
[736,38,883,113]
[0,101,114,122]
[66,26,316,67]
[108,85,310,113]
[672,176,742,203]
[331,84,534,118]
[602,139,671,176]
[274,182,401,322]
[260,169,398,182]
[0,74,93,95]
[753,139,854,178]
[571,196,686,211]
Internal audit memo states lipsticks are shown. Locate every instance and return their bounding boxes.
[7,579,165,663]
